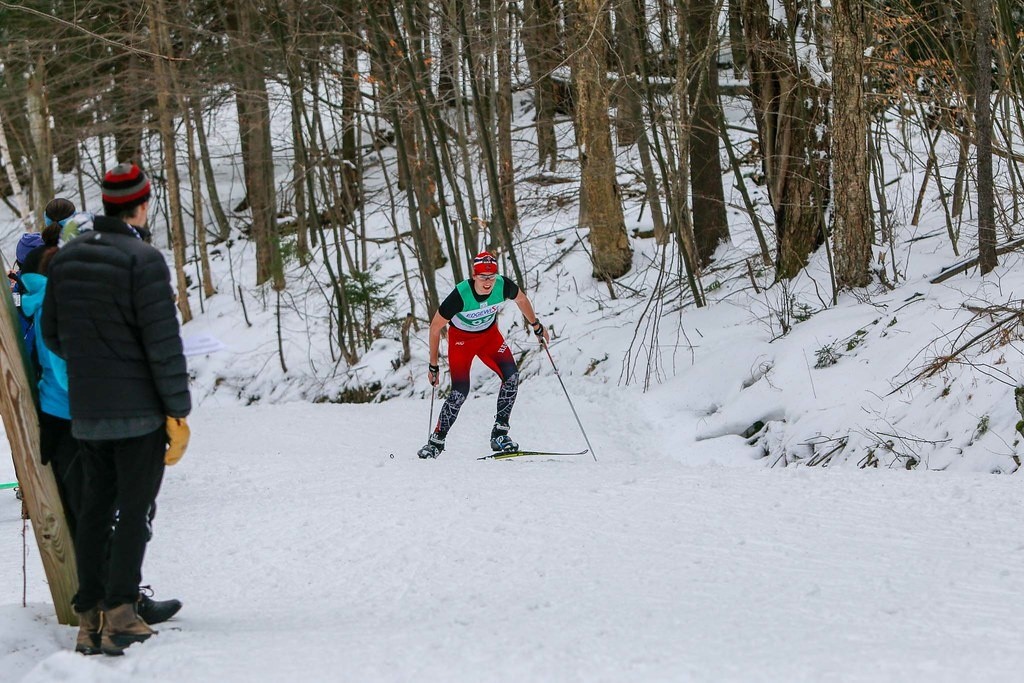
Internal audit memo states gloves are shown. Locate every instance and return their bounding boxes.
[165,414,191,466]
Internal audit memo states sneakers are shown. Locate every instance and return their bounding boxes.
[417,434,445,459]
[135,585,183,625]
[490,426,519,452]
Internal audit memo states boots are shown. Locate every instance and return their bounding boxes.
[97,601,182,656]
[75,607,101,656]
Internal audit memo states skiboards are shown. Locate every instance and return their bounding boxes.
[476,449,588,461]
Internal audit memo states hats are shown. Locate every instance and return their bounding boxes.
[473,252,499,275]
[101,163,150,204]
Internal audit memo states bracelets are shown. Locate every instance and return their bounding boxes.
[534,323,543,334]
[531,318,539,326]
[429,363,439,372]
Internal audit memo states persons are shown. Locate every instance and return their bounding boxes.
[417,251,549,458]
[5,160,192,654]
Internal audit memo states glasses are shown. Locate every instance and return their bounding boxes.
[476,273,497,281]
